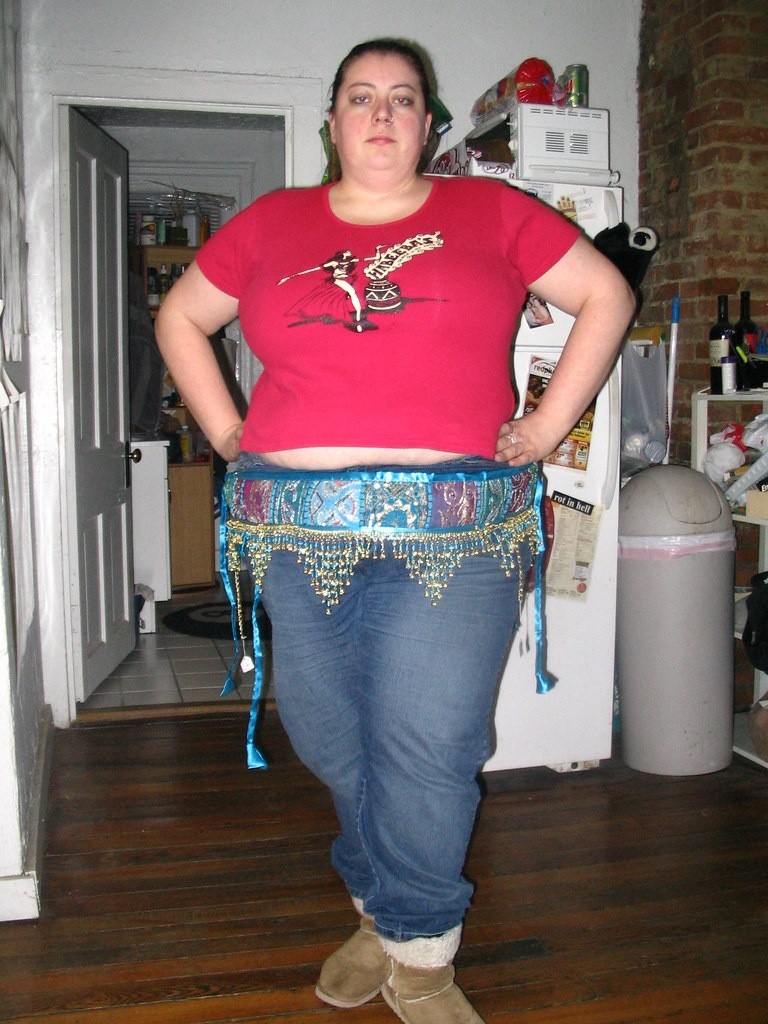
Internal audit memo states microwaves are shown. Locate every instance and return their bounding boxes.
[461,102,612,189]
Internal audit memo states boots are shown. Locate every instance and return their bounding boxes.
[379,922,486,1024]
[315,896,392,1008]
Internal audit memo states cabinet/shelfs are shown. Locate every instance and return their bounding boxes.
[127,245,214,593]
[690,388,768,769]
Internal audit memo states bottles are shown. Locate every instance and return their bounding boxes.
[707,290,736,394]
[147,261,182,306]
[720,356,737,395]
[183,209,200,248]
[200,215,211,247]
[734,290,758,392]
[181,426,193,463]
[140,214,157,245]
[157,219,166,244]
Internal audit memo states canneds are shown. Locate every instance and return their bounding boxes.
[557,64,588,107]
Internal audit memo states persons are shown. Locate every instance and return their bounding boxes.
[155,37,636,1024]
[524,297,553,327]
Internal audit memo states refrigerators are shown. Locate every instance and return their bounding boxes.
[395,174,627,777]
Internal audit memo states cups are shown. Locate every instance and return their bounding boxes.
[165,228,188,246]
[748,357,768,389]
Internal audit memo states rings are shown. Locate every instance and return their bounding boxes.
[508,435,517,443]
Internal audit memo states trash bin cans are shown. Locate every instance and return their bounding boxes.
[619,463,740,777]
